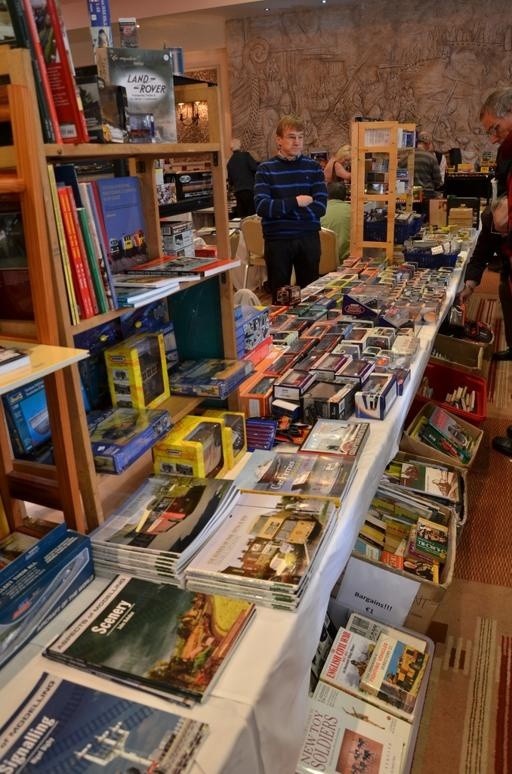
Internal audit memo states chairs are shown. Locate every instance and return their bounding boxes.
[239,219,266,289]
[319,227,338,276]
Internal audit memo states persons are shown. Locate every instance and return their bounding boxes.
[320,145,352,261]
[479,91,512,145]
[491,170,512,462]
[253,116,328,302]
[455,202,512,363]
[397,132,446,221]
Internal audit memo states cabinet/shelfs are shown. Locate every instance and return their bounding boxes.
[349,121,421,266]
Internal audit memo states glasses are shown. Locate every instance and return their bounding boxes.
[488,118,502,138]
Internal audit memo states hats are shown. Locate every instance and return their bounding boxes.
[326,181,347,200]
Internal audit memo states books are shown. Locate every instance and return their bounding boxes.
[0,574,256,774]
[412,381,474,464]
[352,458,463,582]
[0,0,178,147]
[294,613,430,774]
[66,449,358,612]
[47,165,242,323]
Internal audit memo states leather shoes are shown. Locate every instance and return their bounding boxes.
[494,348,512,361]
[491,436,512,457]
